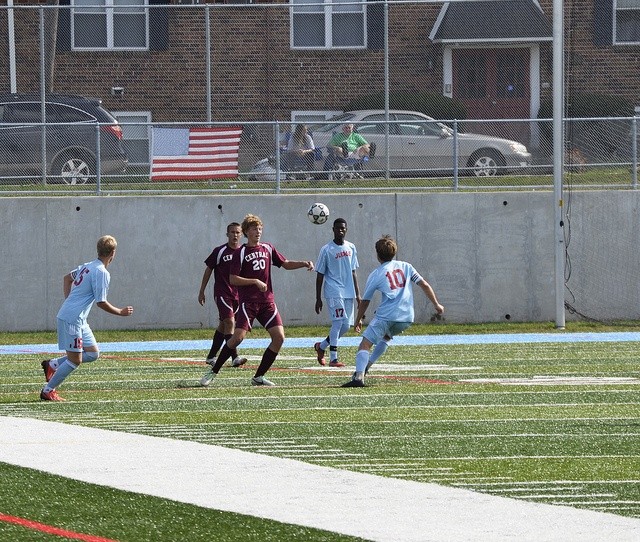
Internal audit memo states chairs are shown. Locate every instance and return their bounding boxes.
[327,131,369,179]
[285,132,314,180]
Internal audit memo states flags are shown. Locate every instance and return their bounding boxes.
[149,126,244,182]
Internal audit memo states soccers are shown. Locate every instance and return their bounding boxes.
[308,203,330,225]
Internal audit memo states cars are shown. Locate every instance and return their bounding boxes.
[249,155,287,180]
[305,109,532,175]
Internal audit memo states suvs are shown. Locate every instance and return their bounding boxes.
[0,92,128,185]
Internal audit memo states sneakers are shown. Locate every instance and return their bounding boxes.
[369,142,376,159]
[315,342,326,366]
[341,380,364,387]
[232,356,247,367]
[40,388,66,401]
[206,357,217,367]
[251,376,275,386]
[41,360,56,382]
[287,175,295,180]
[201,370,216,386]
[341,142,349,158]
[329,359,345,367]
[352,368,368,381]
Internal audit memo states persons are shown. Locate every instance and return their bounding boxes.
[341,238,444,387]
[40,235,134,401]
[199,215,314,387]
[314,218,362,366]
[198,222,248,368]
[326,124,370,180]
[286,123,315,181]
